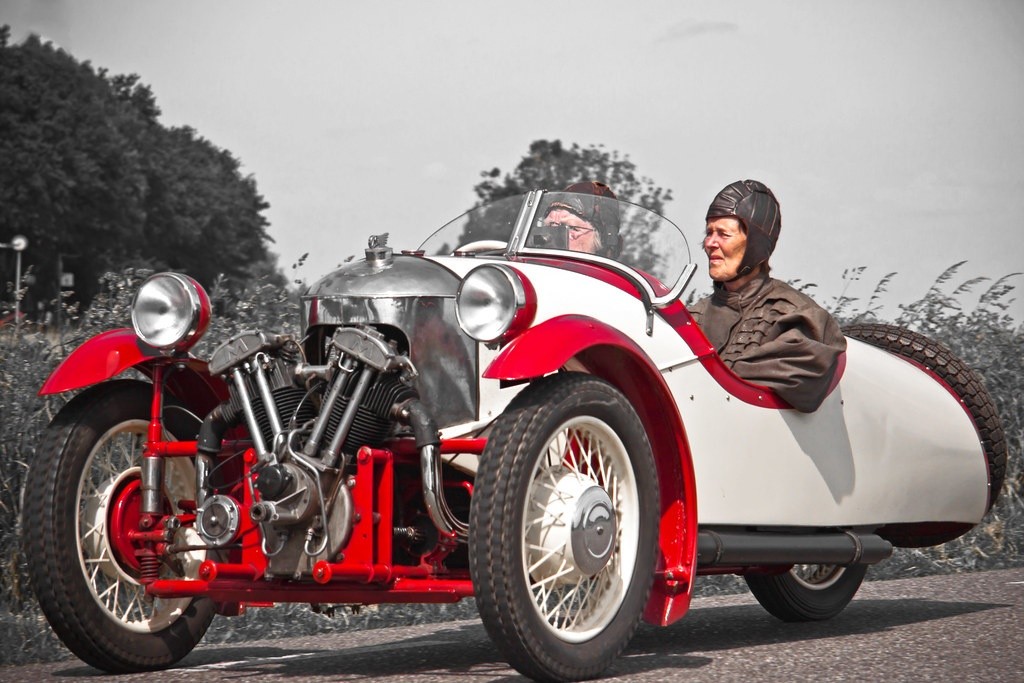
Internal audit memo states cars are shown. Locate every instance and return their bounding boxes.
[20,189,1009,683]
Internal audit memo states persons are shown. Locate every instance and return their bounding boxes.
[542,182,623,260]
[684,180,846,413]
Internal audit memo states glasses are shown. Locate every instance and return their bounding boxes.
[542,221,598,236]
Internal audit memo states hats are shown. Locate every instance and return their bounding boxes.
[706,180,781,277]
[542,181,620,257]
